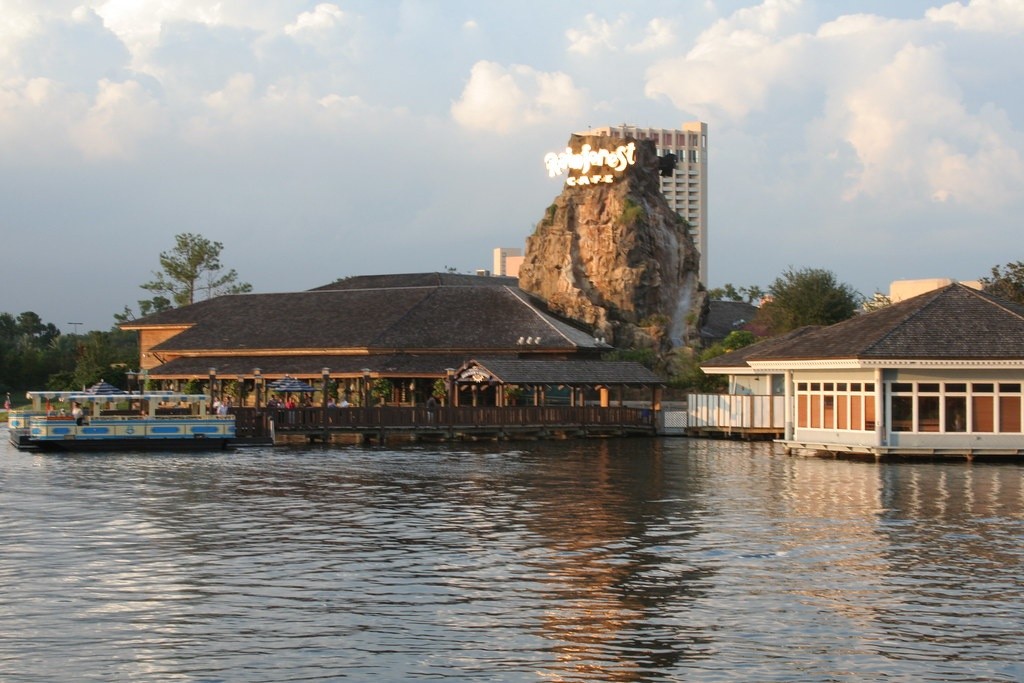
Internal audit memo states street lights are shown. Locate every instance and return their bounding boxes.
[361,367,372,427]
[208,368,216,415]
[252,368,263,437]
[321,366,332,441]
[444,367,457,439]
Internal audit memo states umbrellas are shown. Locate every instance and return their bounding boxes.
[266,374,315,402]
[82,378,127,397]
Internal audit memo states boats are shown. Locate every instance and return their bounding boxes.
[7,389,237,454]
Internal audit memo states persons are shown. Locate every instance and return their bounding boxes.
[48,404,65,416]
[427,392,442,427]
[45,397,50,414]
[72,402,84,426]
[212,395,232,415]
[4,392,11,410]
[327,393,349,408]
[267,393,312,425]
